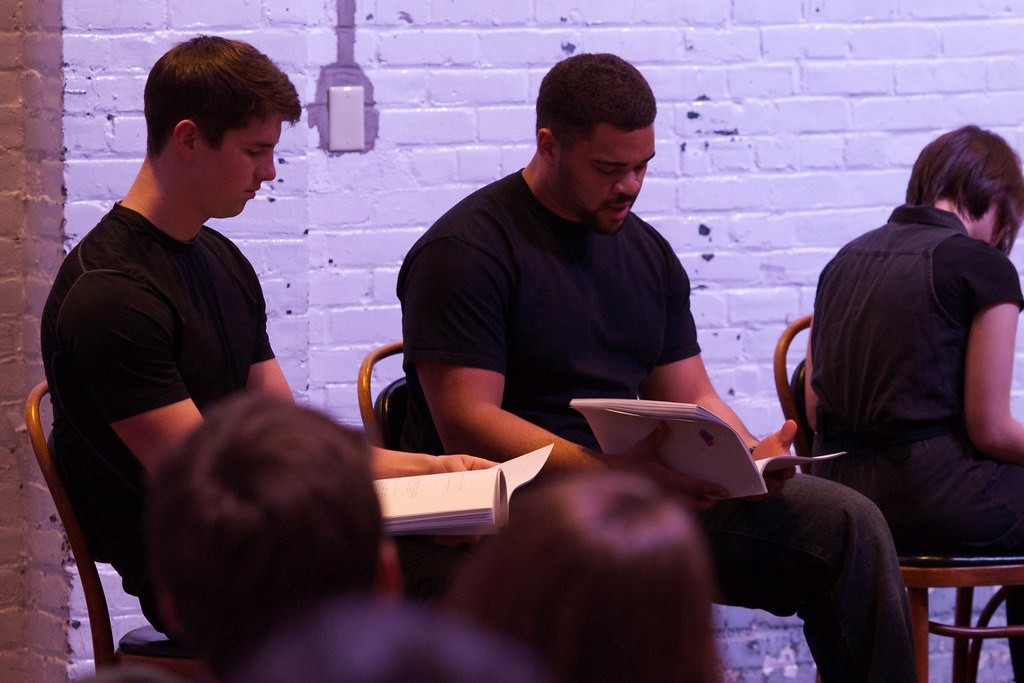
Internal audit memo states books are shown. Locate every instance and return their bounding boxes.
[372,443,554,535]
[571,399,849,498]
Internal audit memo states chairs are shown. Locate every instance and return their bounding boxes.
[22,379,214,683]
[774,313,1024,683]
[357,340,475,617]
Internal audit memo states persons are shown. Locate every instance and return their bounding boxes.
[804,126,1024,683]
[144,398,552,682]
[40,36,506,640]
[397,55,918,683]
